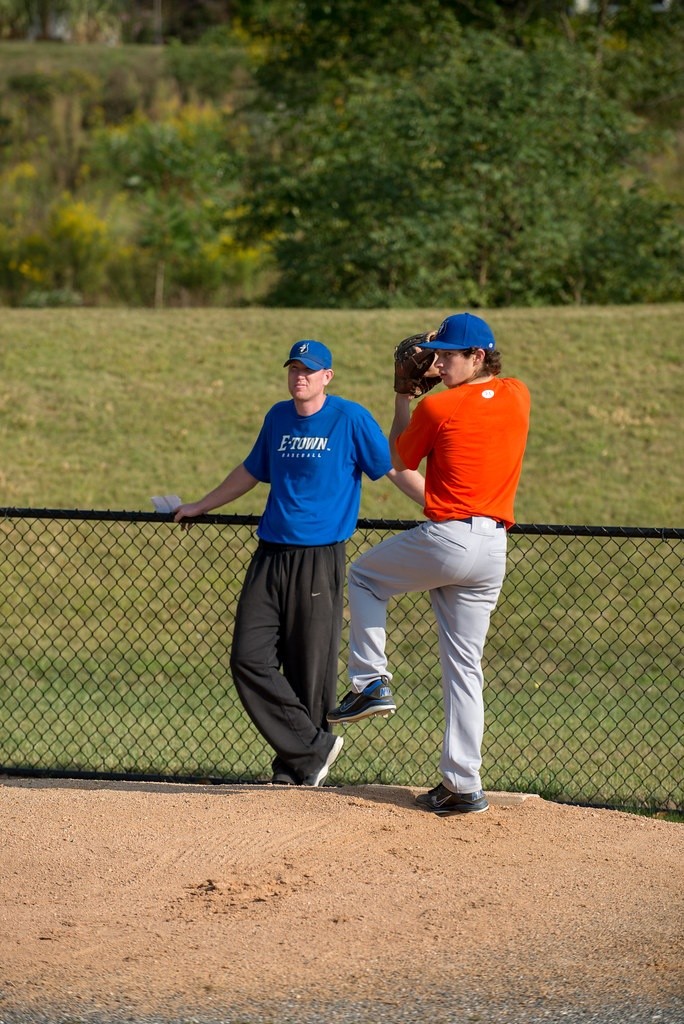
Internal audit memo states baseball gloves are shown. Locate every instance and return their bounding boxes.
[393,329,443,398]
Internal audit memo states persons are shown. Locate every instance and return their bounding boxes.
[325,311,533,816]
[168,338,427,788]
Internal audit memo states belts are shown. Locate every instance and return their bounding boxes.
[459,517,504,528]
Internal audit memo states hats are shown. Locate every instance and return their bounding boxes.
[284,340,332,370]
[417,313,495,351]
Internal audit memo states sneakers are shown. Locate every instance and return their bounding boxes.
[326,676,396,725]
[271,780,292,785]
[415,782,489,813]
[302,735,343,787]
